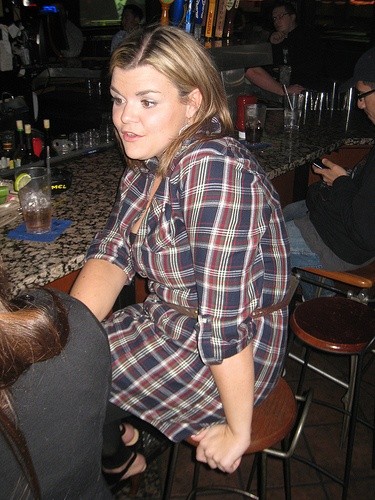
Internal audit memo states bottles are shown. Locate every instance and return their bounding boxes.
[42,118,55,169]
[0,120,39,169]
[235,94,257,139]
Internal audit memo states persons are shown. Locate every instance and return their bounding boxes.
[0,267,112,500]
[223,1,315,96]
[70,24,292,500]
[111,5,144,52]
[282,49,375,376]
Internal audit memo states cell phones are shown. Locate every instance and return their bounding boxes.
[314,160,329,170]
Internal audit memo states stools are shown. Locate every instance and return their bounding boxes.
[162,265,375,500]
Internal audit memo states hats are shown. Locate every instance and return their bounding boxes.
[341,49,375,90]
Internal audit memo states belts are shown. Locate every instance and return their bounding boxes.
[165,293,292,319]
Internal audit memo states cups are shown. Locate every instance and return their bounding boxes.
[283,93,306,129]
[299,79,356,112]
[15,166,51,234]
[71,124,114,151]
[243,104,267,143]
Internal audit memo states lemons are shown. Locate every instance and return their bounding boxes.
[14,173,31,191]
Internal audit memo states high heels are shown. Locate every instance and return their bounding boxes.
[119,422,144,453]
[101,451,148,496]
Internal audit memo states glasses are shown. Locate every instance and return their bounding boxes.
[273,11,292,21]
[357,90,375,102]
